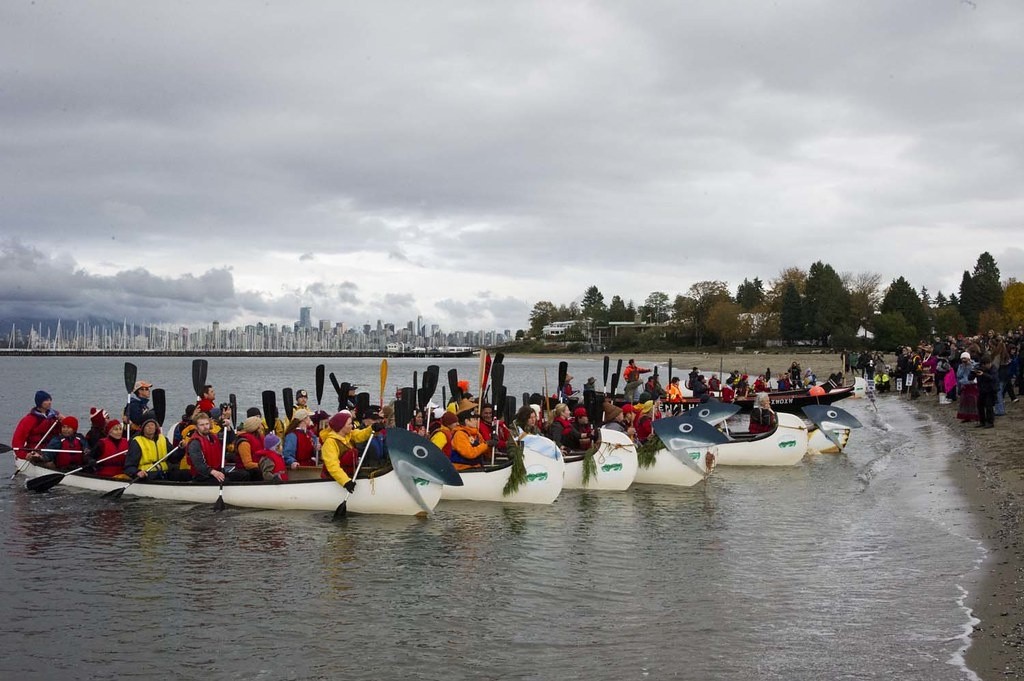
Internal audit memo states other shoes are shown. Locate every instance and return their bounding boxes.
[962,399,1018,428]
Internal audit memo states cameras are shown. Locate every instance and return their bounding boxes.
[220,402,233,411]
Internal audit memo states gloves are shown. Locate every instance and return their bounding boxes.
[345,481,356,493]
[487,436,499,446]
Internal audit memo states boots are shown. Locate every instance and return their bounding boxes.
[939,392,951,404]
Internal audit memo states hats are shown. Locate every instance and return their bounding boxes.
[35,391,52,406]
[587,377,597,383]
[89,407,109,429]
[903,346,912,353]
[243,382,381,450]
[140,416,159,432]
[105,419,120,434]
[575,409,589,416]
[427,398,478,426]
[133,381,152,392]
[61,416,78,431]
[742,375,747,379]
[602,400,653,423]
[565,374,573,382]
[960,352,971,359]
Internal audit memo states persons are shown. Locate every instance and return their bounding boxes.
[840,324,1024,428]
[125,419,189,479]
[186,413,234,483]
[120,381,155,437]
[31,416,90,470]
[174,384,231,474]
[12,391,64,461]
[85,407,110,447]
[666,377,683,401]
[83,420,129,477]
[689,367,770,403]
[624,359,653,382]
[318,413,383,493]
[235,382,393,481]
[409,381,509,470]
[260,434,286,481]
[781,361,816,389]
[511,406,540,441]
[751,393,775,430]
[532,374,667,458]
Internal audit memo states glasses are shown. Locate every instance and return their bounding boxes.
[140,388,150,391]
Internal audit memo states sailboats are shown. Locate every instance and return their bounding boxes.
[7,316,377,351]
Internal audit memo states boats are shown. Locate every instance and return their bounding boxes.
[438,426,567,506]
[13,426,464,519]
[495,375,871,488]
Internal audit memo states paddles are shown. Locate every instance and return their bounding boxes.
[10,418,60,478]
[0,442,83,455]
[25,448,127,493]
[117,347,777,469]
[333,428,376,520]
[101,443,183,502]
[212,421,228,513]
